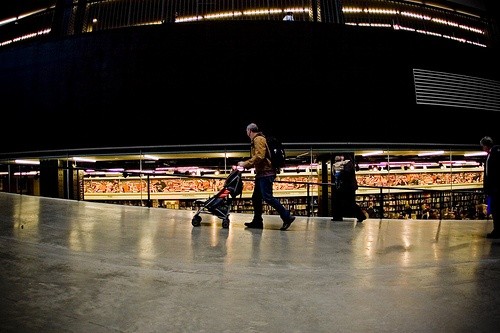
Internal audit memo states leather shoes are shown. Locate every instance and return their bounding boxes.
[279,216,295,231]
[244,219,263,229]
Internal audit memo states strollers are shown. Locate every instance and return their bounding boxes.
[190,163,249,228]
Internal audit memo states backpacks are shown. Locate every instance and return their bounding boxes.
[251,134,286,173]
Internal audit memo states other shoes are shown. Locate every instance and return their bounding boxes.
[330,218,343,222]
[357,217,367,222]
[486,230,500,239]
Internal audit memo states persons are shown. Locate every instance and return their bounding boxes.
[332,156,350,185]
[331,154,367,223]
[479,136,500,239]
[238,123,295,231]
